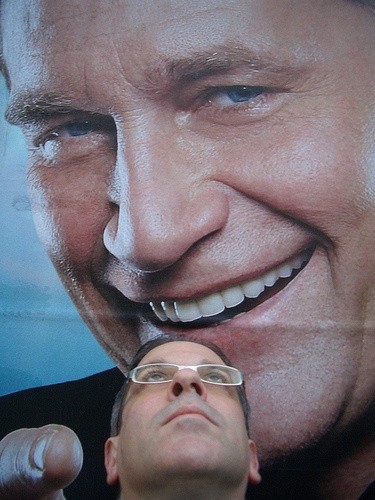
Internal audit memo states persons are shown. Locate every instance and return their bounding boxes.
[103,333,260,500]
[0,1,375,500]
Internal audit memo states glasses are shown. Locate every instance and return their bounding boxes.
[126,363,249,406]
